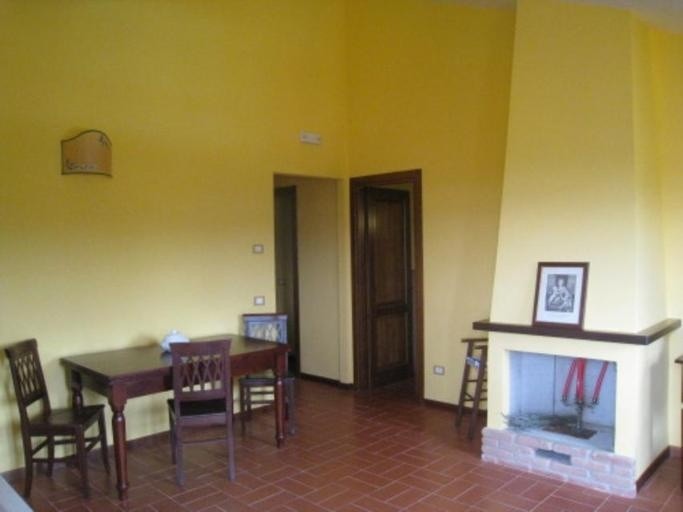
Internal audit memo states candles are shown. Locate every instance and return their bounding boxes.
[562,357,608,402]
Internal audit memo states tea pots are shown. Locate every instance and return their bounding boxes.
[161,329,191,353]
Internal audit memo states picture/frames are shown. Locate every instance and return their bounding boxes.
[533,261,588,330]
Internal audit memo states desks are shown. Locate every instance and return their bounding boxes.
[58,333,294,500]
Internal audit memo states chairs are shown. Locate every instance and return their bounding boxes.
[4,337,112,499]
[166,338,236,488]
[237,310,288,435]
[457,338,490,435]
